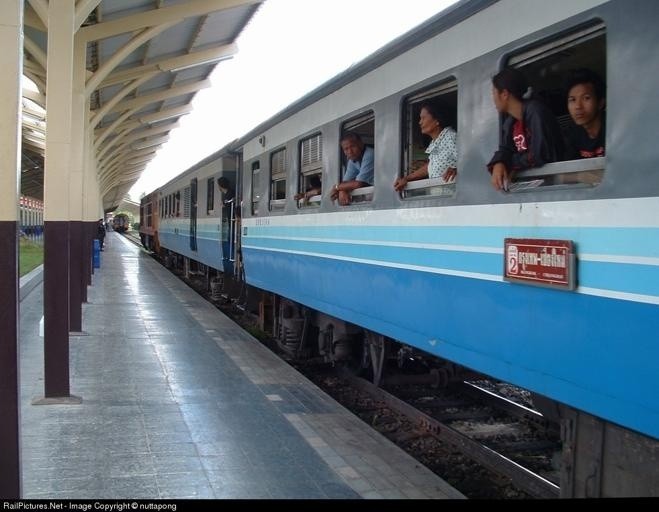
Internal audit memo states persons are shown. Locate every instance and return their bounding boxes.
[393,100,458,196]
[98,218,106,250]
[294,174,322,207]
[216,177,237,258]
[234,205,241,222]
[329,131,374,206]
[552,72,606,188]
[485,65,560,195]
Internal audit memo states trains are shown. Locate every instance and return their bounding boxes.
[139,0,658,499]
[112,213,129,232]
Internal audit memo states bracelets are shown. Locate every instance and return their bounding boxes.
[336,183,339,191]
